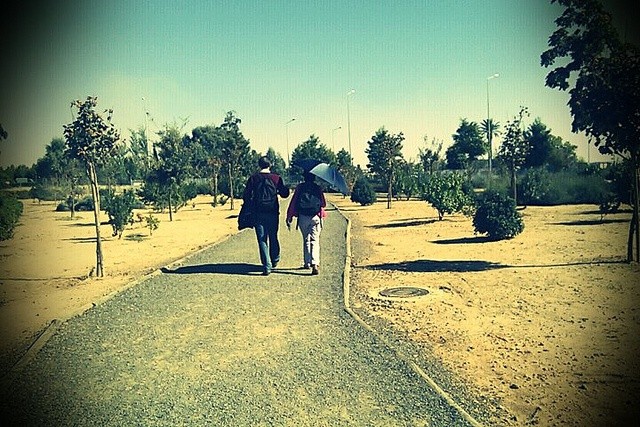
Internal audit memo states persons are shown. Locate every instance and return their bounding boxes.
[243,156,289,276]
[286,169,326,275]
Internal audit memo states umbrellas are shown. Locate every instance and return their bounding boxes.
[291,158,349,195]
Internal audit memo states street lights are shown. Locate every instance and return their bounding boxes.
[286,118,295,167]
[347,88,356,165]
[141,96,150,168]
[486,73,500,173]
[332,126,342,153]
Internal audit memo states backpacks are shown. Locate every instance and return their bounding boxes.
[298,183,321,215]
[254,174,277,212]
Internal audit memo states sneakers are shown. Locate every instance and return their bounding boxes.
[263,270,270,275]
[304,263,310,268]
[312,265,319,275]
